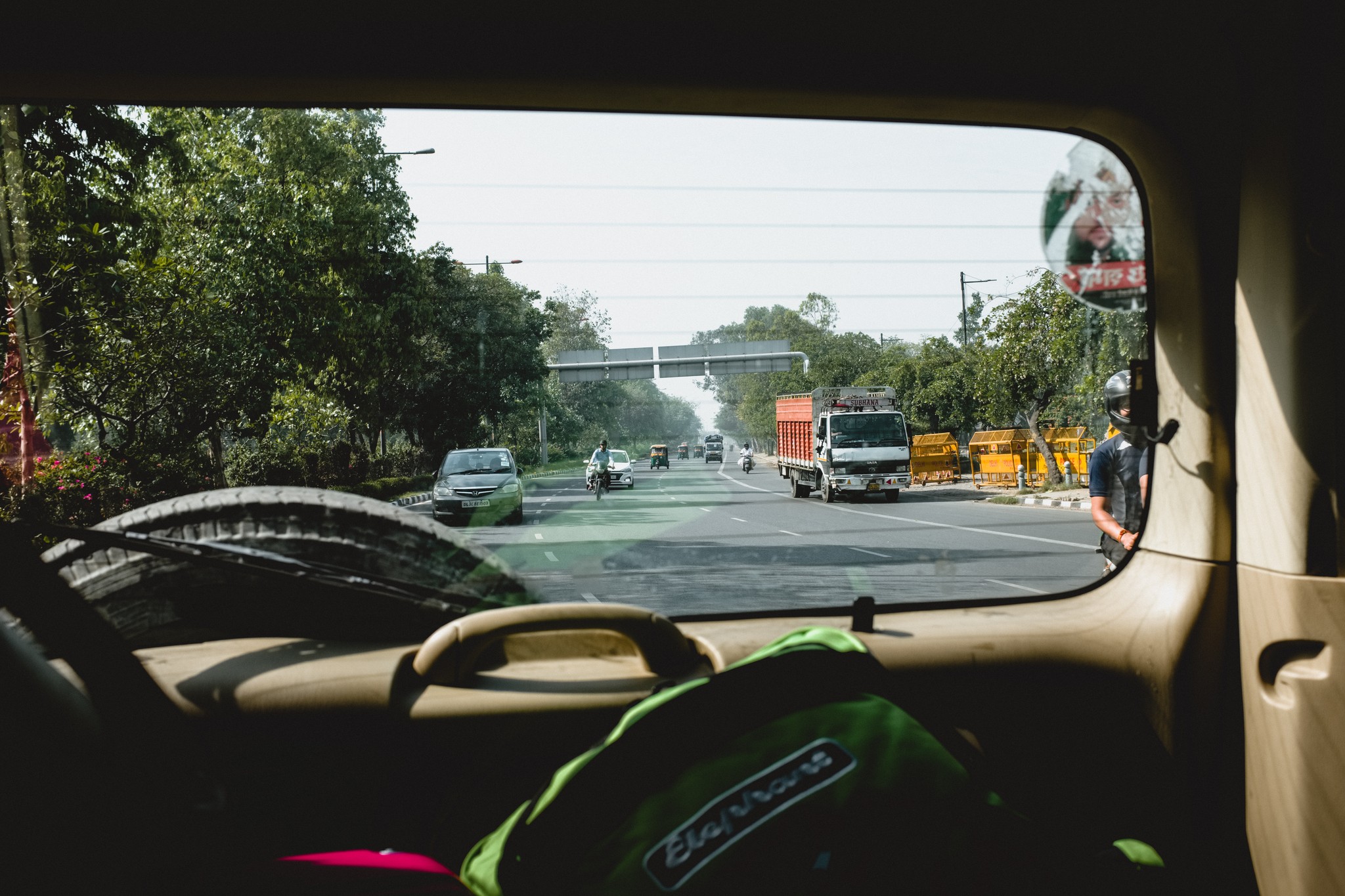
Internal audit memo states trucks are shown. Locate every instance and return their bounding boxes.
[703,434,723,464]
[774,386,912,503]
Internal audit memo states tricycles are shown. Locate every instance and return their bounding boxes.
[729,444,733,453]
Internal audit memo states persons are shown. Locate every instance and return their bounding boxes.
[456,454,471,470]
[879,417,900,439]
[831,419,843,437]
[587,440,615,494]
[490,457,502,471]
[739,442,754,472]
[1137,446,1149,507]
[1088,370,1152,572]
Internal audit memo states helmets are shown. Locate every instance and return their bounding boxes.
[744,442,749,447]
[1103,369,1147,436]
[599,440,607,444]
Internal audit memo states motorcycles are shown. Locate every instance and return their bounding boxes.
[649,444,670,470]
[588,461,615,500]
[693,445,704,458]
[677,444,689,460]
[740,454,754,474]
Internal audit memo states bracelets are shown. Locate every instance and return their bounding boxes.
[1117,530,1133,544]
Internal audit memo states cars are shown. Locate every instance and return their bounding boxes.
[583,449,637,490]
[430,447,525,527]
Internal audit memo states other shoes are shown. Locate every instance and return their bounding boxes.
[588,486,593,490]
[605,487,609,494]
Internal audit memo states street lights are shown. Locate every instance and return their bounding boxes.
[371,148,436,460]
[484,256,523,276]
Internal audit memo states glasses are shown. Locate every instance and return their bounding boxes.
[1119,401,1132,412]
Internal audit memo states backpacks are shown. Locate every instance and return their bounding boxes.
[460,623,1169,895]
